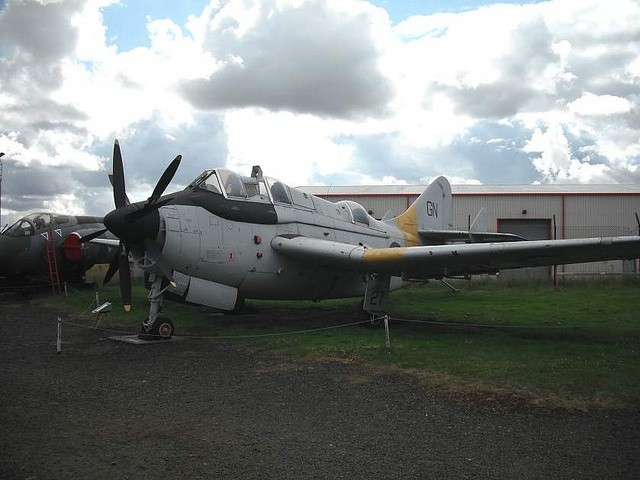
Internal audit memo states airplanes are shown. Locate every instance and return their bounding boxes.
[0,211,142,283]
[78,137,639,341]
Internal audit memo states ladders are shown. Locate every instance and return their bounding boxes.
[46,229,61,294]
